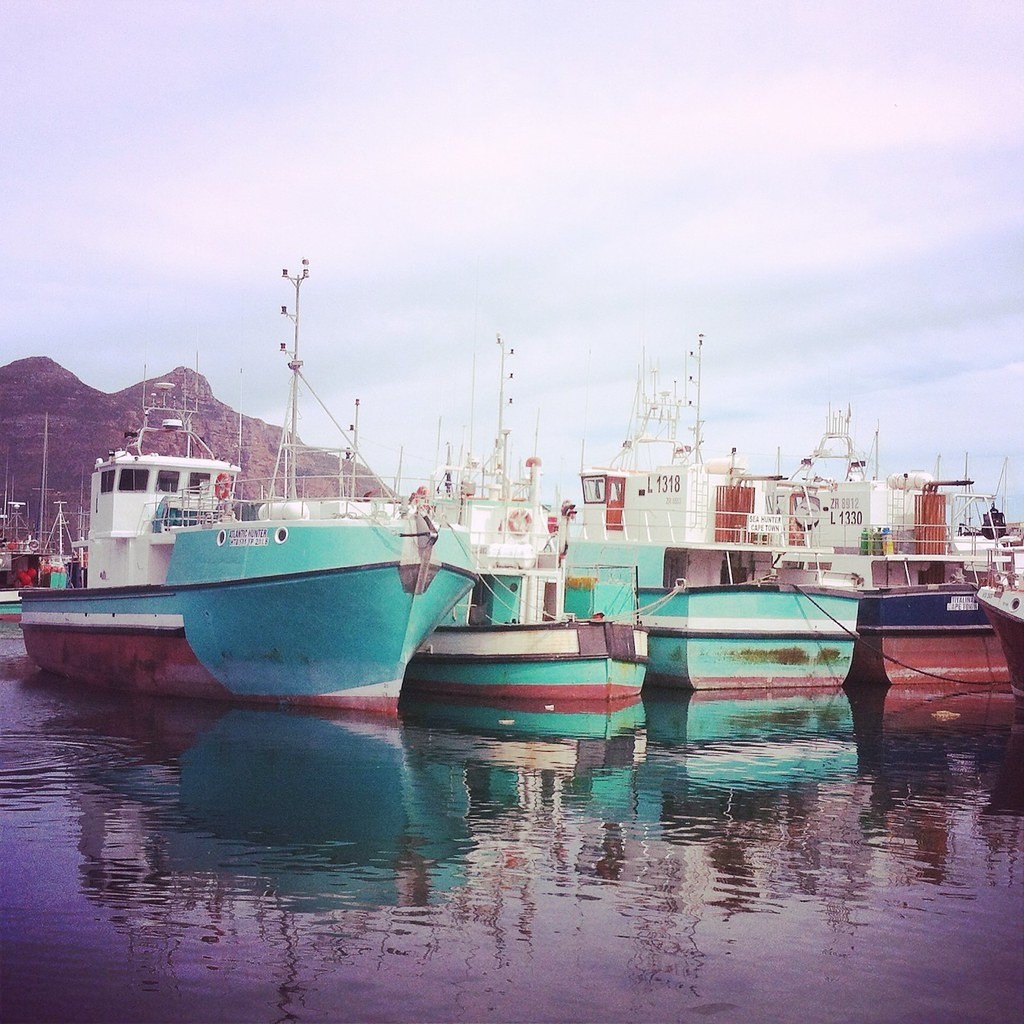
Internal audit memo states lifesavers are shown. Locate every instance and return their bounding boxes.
[509,509,531,537]
[29,539,39,551]
[416,486,429,509]
[214,472,230,500]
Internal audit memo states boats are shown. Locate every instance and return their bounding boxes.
[401,330,653,702]
[640,685,862,857]
[21,668,473,1023]
[844,679,1017,883]
[15,256,481,720]
[0,322,1024,718]
[398,689,650,969]
